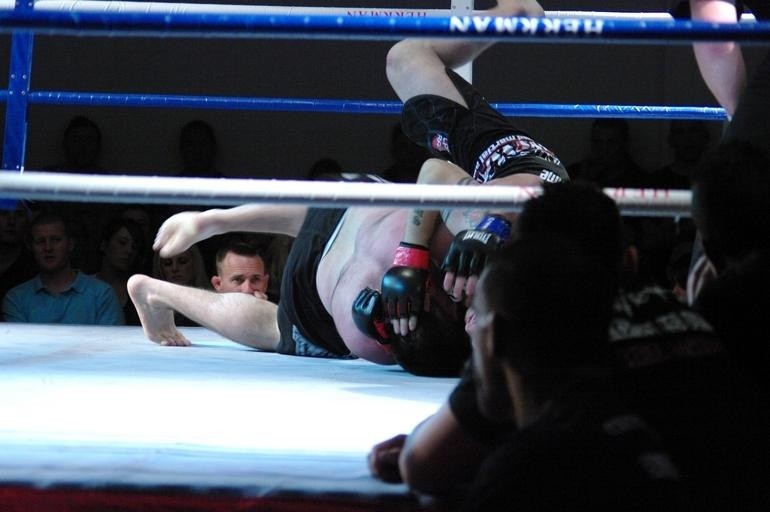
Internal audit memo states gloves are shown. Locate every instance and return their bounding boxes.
[441,213,513,278]
[382,241,435,339]
[351,286,402,359]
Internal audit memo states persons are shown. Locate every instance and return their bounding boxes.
[0,1,770,512]
